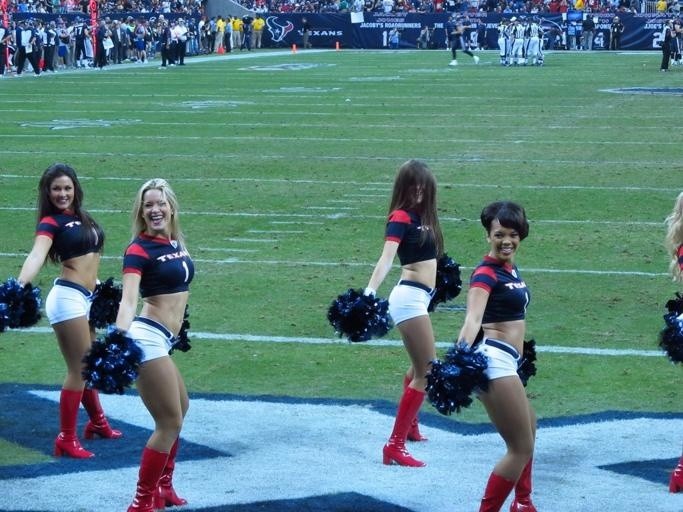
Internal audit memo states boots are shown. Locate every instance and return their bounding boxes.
[53,388,96,458]
[82,387,123,439]
[480,456,540,511]
[404,374,428,441]
[126,436,188,511]
[383,387,427,466]
[670,455,683,491]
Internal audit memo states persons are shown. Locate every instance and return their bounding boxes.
[657,186,683,494]
[111,177,197,512]
[450,200,545,512]
[363,157,447,468]
[0,0,682,79]
[12,162,122,461]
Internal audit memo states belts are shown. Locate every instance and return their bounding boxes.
[132,316,181,345]
[486,340,529,369]
[399,281,437,297]
[56,279,98,301]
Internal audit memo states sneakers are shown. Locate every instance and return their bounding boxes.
[450,60,458,66]
[474,56,479,64]
